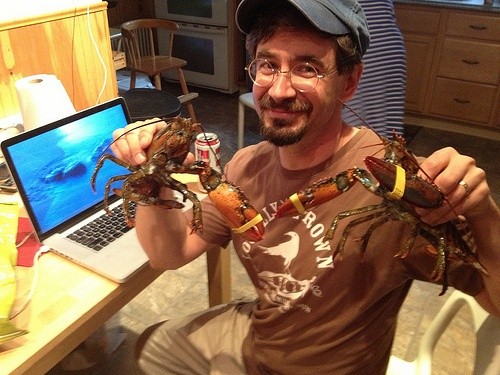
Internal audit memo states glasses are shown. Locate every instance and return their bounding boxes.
[245,57,335,92]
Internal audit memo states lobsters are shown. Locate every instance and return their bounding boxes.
[84,112,268,245]
[273,95,495,300]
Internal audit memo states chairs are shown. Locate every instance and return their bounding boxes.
[238,92,255,149]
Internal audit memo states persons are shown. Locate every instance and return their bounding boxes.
[111,0,500,374]
[342,0,406,139]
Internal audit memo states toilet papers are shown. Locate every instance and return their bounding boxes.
[14,75,76,131]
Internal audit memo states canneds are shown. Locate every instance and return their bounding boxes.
[194,133,222,174]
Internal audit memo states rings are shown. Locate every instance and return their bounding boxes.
[460,181,469,194]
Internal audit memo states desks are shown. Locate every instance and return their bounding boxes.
[1,173,232,375]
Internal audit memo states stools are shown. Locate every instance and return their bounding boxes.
[120,18,194,117]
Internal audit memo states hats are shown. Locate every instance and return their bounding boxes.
[236,0,371,56]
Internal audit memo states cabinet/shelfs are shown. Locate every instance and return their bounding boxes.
[393,4,500,141]
